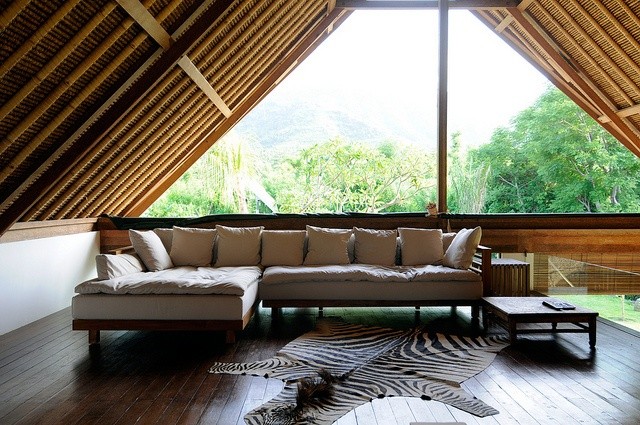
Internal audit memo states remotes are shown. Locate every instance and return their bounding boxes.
[543,300,563,310]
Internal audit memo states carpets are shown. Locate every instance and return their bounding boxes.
[209,322,515,424]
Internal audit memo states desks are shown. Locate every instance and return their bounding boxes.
[481,296,599,347]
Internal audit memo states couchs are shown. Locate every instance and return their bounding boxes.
[169,225,218,267]
[70,224,492,346]
[261,230,307,268]
[214,225,265,268]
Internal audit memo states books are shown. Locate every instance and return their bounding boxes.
[543,299,577,309]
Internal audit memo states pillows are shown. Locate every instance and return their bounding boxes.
[303,225,353,266]
[128,229,174,272]
[398,228,444,266]
[352,226,397,266]
[442,226,482,270]
